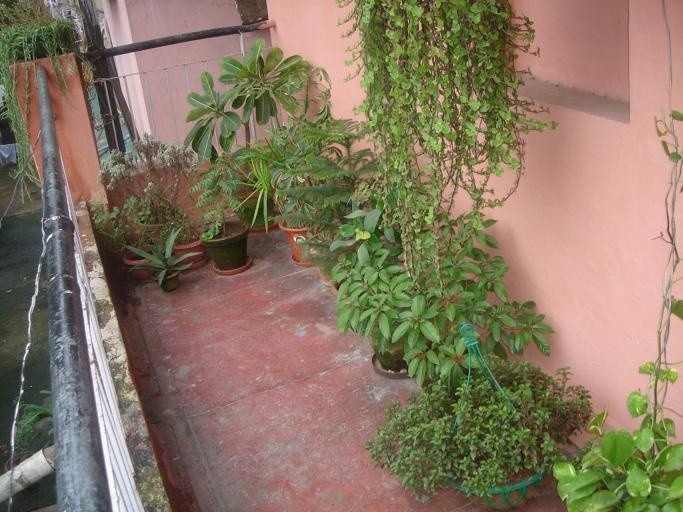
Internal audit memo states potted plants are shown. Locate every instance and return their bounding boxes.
[90,131,253,289]
[185,39,339,266]
[262,116,591,511]
